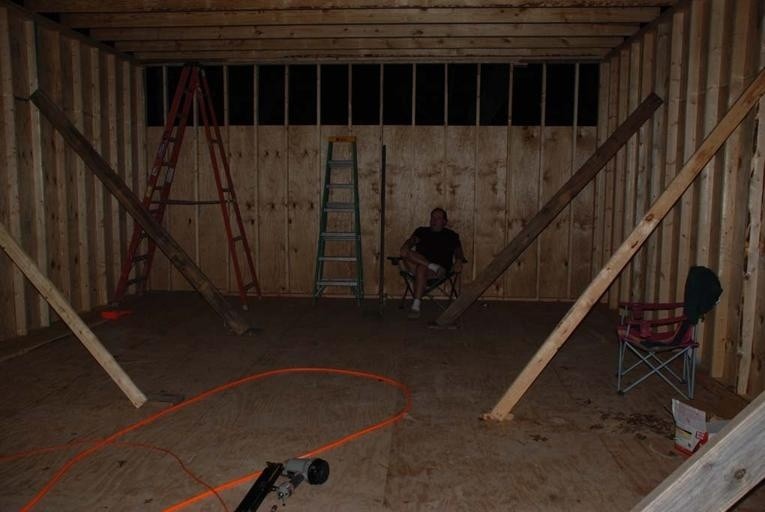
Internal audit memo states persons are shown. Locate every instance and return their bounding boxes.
[399,207,463,320]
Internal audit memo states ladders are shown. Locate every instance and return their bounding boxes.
[112,61,262,311]
[312,136,363,307]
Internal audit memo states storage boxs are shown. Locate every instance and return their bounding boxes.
[671,397,731,456]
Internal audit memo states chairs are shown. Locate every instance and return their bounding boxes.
[617,266,700,401]
[389,253,468,310]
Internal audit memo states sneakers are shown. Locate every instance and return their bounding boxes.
[435,266,446,283]
[408,308,423,320]
